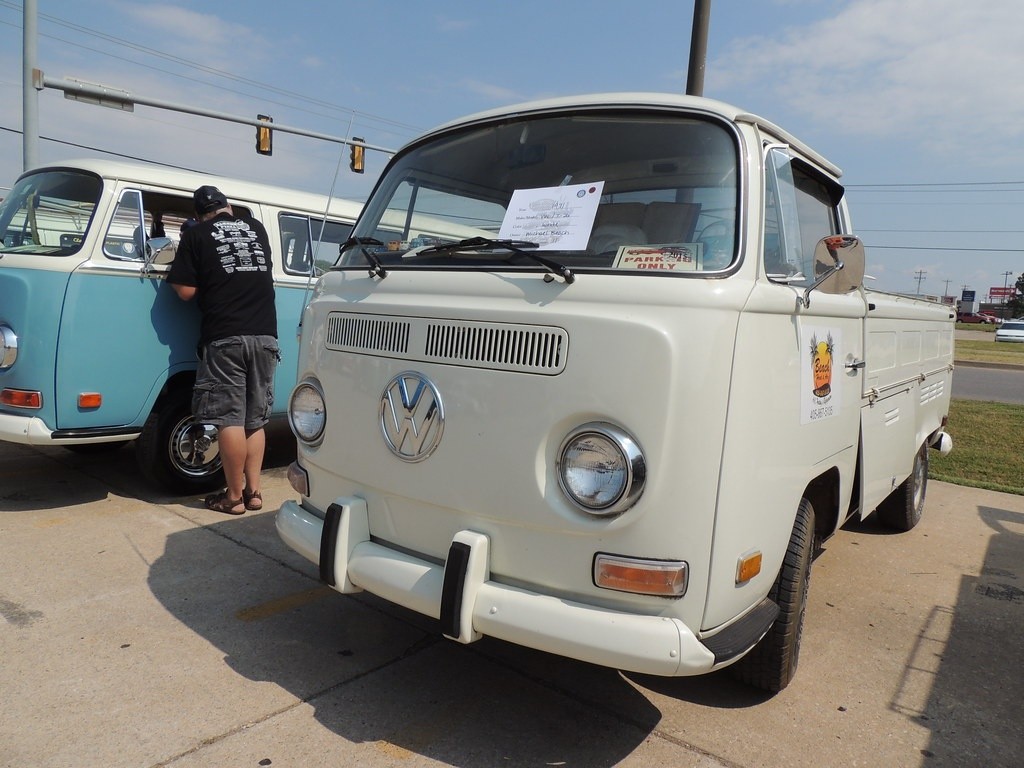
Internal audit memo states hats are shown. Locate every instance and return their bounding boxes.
[180,218,199,231]
[193,186,227,214]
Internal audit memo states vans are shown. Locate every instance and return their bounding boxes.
[0,159,502,495]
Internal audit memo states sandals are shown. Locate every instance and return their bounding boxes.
[243,488,263,510]
[205,489,246,515]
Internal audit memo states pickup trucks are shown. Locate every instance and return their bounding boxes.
[275,93,954,693]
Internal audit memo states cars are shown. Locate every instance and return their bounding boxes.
[980,313,997,324]
[956,312,988,324]
[994,322,1024,343]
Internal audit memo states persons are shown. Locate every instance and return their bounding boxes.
[169,185,278,515]
